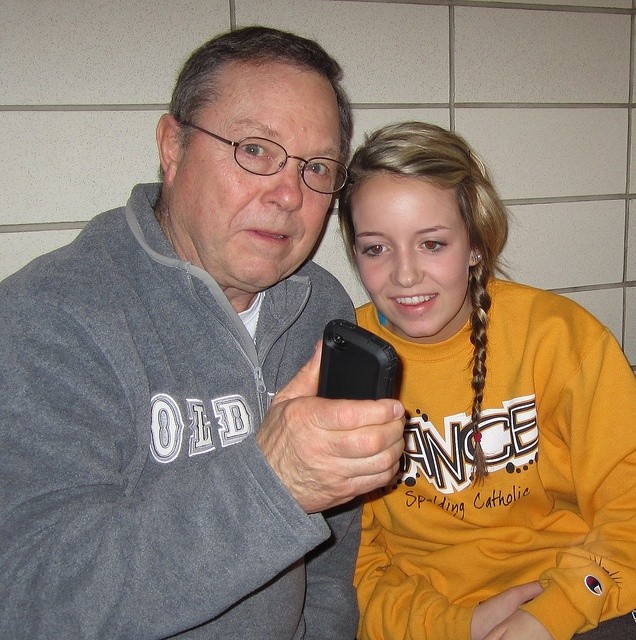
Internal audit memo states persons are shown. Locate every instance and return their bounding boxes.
[1,24,407,640]
[334,122,636,638]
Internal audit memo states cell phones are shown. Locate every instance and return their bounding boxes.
[316,318,399,400]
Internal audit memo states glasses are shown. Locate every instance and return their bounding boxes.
[179,120,355,193]
